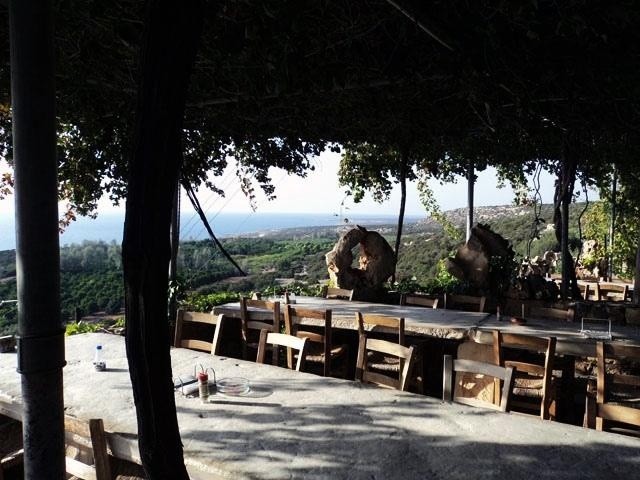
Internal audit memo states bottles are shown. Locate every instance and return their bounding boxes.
[290,293,296,304]
[198,373,209,401]
[93,345,107,372]
[496,305,503,321]
[284,289,290,304]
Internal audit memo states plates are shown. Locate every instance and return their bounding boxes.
[216,378,251,395]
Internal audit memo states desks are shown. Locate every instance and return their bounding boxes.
[1,332,639,479]
[213,294,639,358]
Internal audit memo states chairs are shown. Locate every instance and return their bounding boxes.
[0,273,640,480]
